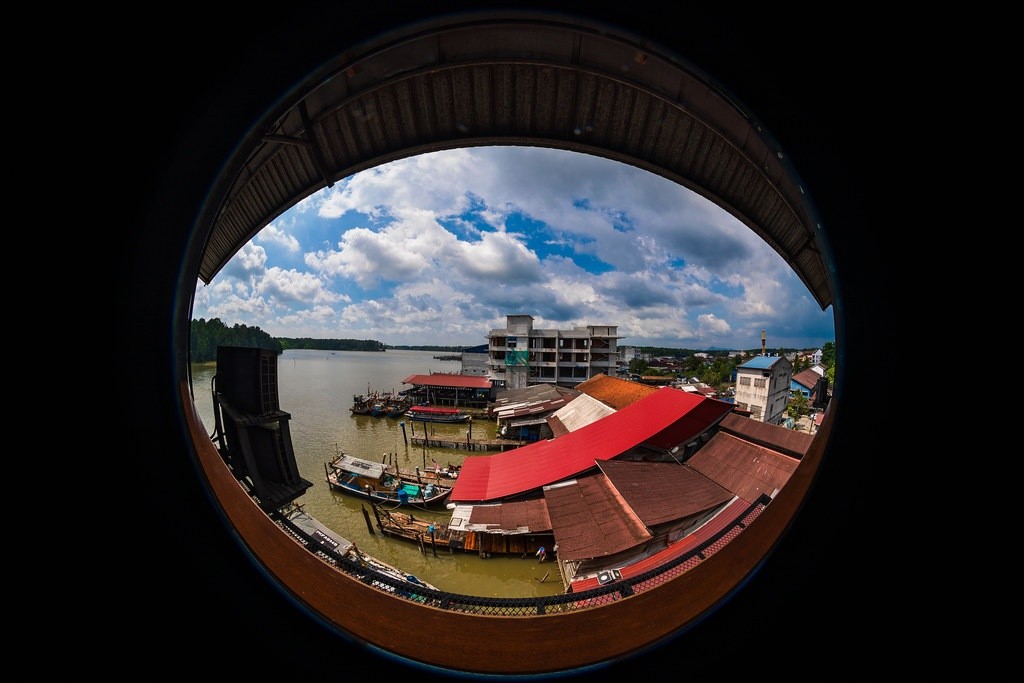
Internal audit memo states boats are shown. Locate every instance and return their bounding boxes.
[324,443,454,511]
[348,381,409,419]
[404,405,471,423]
